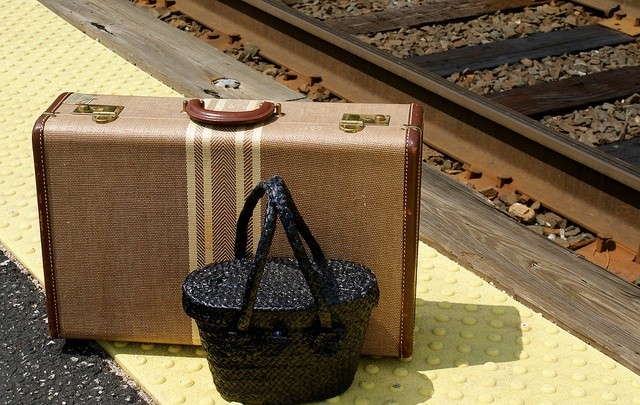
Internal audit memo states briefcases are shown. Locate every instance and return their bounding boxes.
[32,92,425,358]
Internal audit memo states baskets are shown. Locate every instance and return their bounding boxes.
[181,175,379,404]
